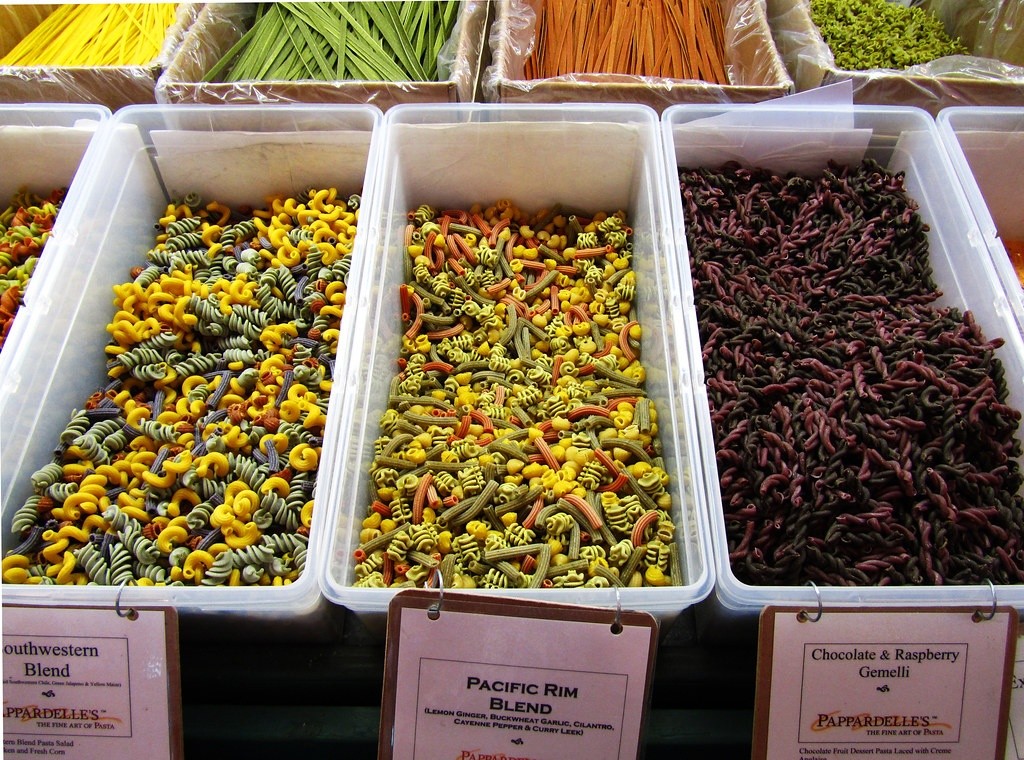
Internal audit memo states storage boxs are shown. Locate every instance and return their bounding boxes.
[0,0,1023,645]
